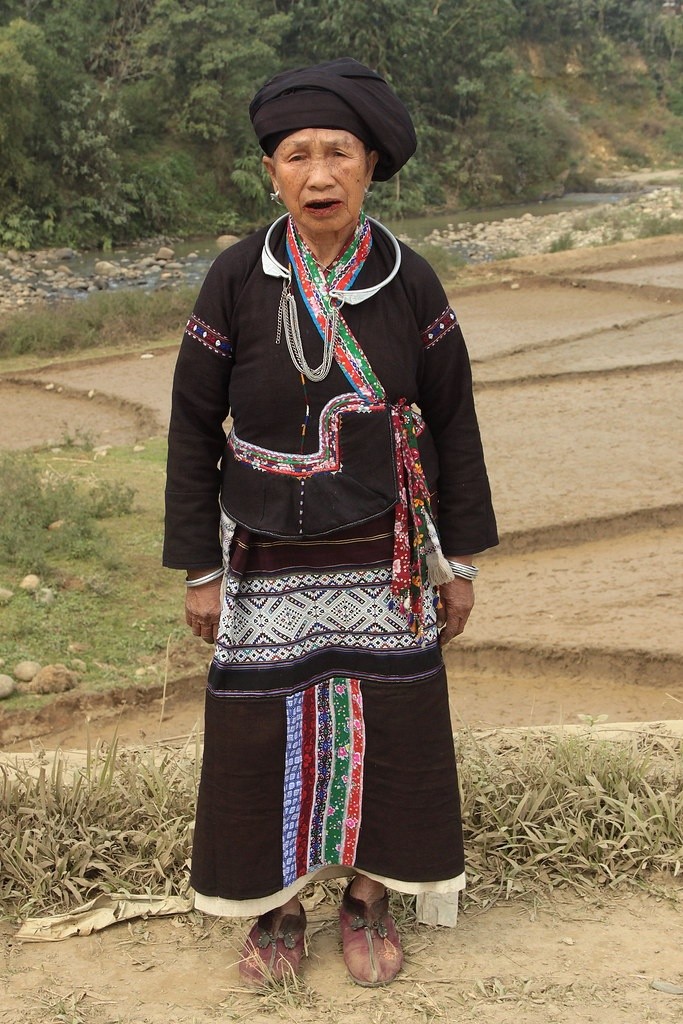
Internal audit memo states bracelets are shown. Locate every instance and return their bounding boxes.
[185,567,225,587]
[447,560,479,581]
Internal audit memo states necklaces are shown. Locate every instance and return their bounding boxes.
[262,212,401,382]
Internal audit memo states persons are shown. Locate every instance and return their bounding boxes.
[162,57,501,990]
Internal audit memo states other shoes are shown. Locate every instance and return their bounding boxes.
[340,877,403,986]
[240,901,307,987]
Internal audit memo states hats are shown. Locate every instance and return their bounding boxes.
[250,57,417,181]
